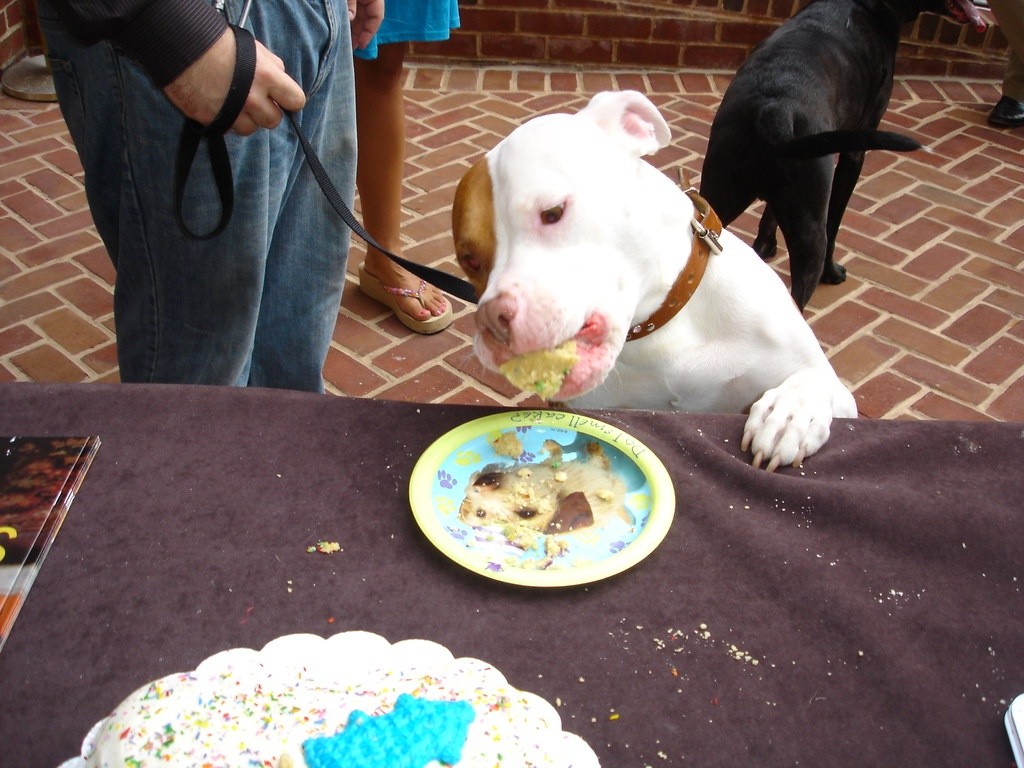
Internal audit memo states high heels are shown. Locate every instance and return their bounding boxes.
[358,261,452,334]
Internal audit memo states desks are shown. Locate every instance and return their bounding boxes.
[0,379,1024,768]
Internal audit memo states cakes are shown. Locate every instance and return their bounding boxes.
[80,656,579,768]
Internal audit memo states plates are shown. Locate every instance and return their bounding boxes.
[409,410,677,587]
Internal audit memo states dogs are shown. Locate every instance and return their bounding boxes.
[456,435,636,538]
[451,87,862,473]
[697,0,987,315]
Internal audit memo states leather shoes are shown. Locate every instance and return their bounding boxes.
[988,95,1024,127]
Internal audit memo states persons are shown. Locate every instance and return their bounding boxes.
[354,0,461,334]
[22,1,384,395]
[987,0,1024,126]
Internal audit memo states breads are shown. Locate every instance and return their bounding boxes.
[501,339,580,401]
